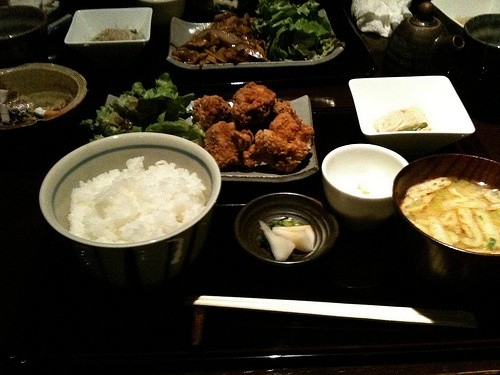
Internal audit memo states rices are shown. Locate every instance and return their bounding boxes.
[66,155,207,244]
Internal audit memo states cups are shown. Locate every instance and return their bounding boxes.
[144,0,185,24]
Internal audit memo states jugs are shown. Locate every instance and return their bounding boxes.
[386,1,465,64]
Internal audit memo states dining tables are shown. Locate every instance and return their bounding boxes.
[0,0,500,375]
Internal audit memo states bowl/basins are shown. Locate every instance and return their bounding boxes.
[0,6,47,41]
[320,144,409,222]
[233,192,339,266]
[393,153,500,280]
[64,6,154,54]
[464,14,500,50]
[1,62,88,132]
[39,132,223,251]
[347,75,476,153]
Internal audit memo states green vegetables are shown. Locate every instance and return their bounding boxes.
[251,0,345,60]
[79,69,206,145]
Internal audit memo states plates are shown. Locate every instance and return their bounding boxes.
[105,93,319,182]
[166,16,343,70]
